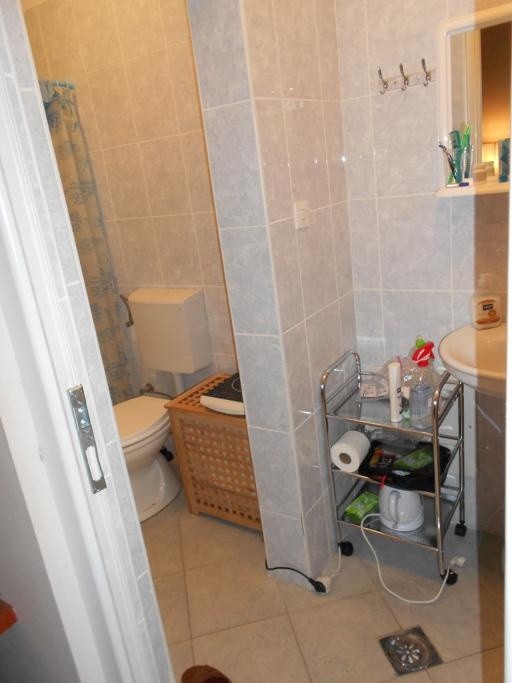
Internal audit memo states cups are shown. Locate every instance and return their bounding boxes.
[441,147,475,188]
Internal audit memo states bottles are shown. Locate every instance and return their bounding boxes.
[388,362,404,422]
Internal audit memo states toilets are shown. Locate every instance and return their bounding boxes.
[110,287,210,525]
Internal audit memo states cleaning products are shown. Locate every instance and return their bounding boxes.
[409,336,436,429]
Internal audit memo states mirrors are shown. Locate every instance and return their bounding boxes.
[438,5,512,199]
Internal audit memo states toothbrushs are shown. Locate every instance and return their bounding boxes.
[436,139,460,183]
[447,123,471,182]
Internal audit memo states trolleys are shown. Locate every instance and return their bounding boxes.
[322,346,468,586]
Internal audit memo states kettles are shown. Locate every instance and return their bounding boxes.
[378,484,426,532]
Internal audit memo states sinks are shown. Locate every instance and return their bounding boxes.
[440,325,506,395]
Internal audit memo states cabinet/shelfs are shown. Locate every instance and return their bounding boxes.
[164,374,264,542]
